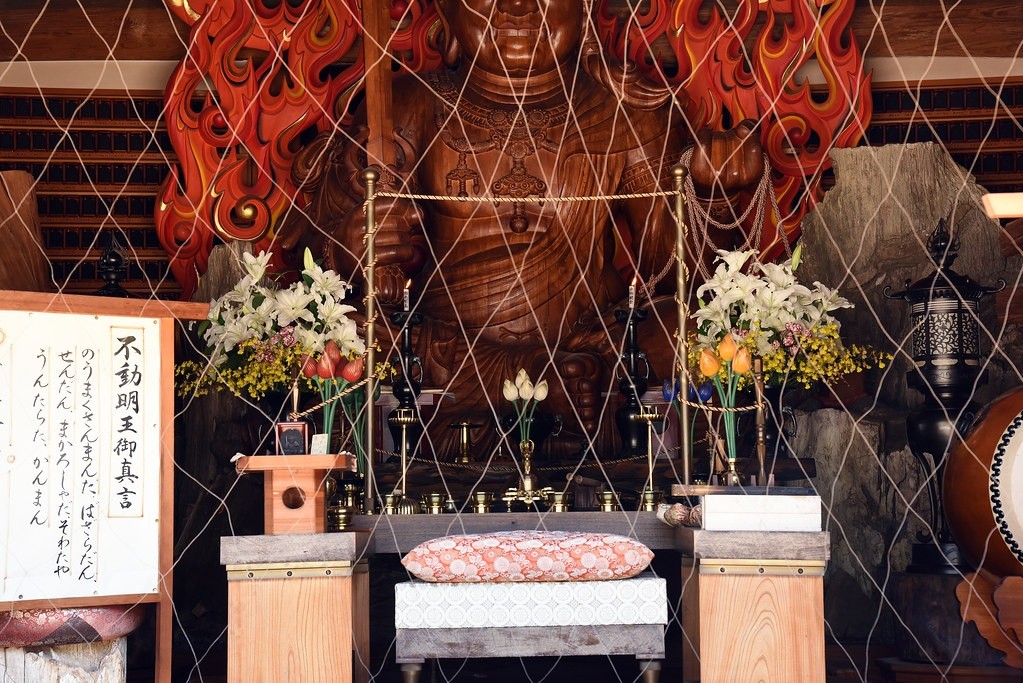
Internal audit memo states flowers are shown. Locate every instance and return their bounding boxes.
[696,241,894,389]
[503,368,549,439]
[178,246,367,395]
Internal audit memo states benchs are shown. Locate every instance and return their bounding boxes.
[394,572,669,683]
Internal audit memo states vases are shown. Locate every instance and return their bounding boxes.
[277,363,309,454]
[520,440,534,490]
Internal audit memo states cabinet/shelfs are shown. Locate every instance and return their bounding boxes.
[674,527,830,683]
[226,559,370,683]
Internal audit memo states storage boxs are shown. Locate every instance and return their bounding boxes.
[700,495,821,532]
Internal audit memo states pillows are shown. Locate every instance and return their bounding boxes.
[401,529,654,582]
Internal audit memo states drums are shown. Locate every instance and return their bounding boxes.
[941,384,1023,576]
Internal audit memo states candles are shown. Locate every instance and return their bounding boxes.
[628,277,637,307]
[403,278,411,311]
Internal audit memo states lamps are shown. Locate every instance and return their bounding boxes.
[882,217,1007,574]
